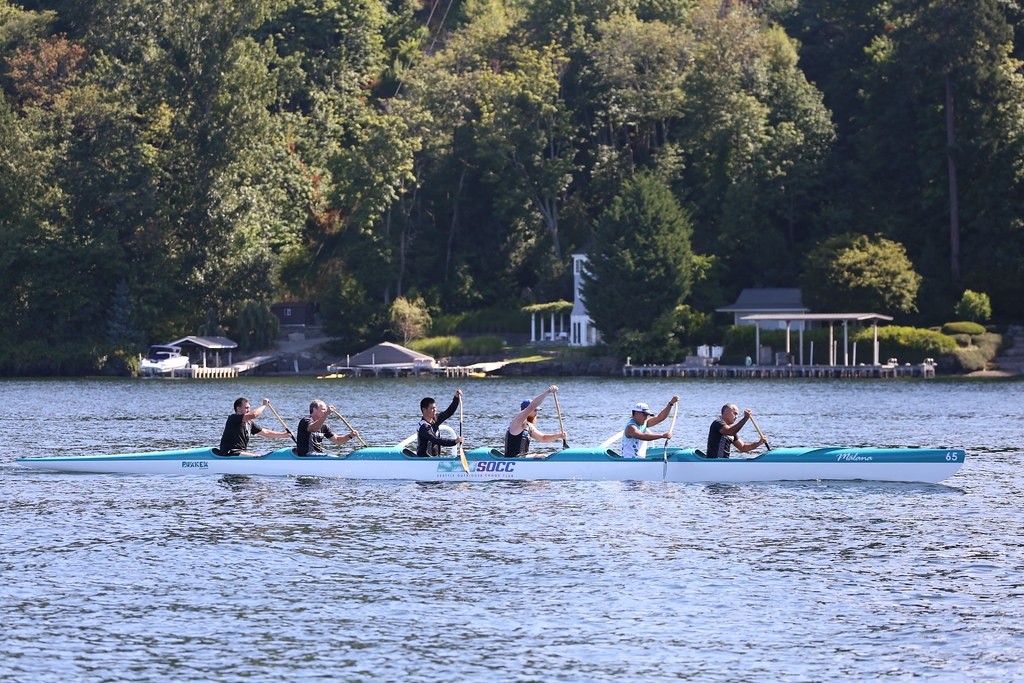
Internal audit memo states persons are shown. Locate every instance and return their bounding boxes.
[504,385,566,458]
[621,395,680,459]
[297,399,358,458]
[417,388,464,458]
[219,398,292,458]
[706,403,768,458]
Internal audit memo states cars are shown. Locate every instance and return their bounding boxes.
[138,350,191,376]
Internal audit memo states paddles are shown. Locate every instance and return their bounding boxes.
[266,400,298,444]
[553,392,568,448]
[457,394,472,474]
[749,414,771,450]
[331,409,366,447]
[663,401,678,463]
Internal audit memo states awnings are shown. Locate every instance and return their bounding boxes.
[739,313,894,368]
[172,335,238,368]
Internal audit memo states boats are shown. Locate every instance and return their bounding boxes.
[11,425,967,487]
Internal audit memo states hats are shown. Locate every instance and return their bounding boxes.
[520,399,542,411]
[632,403,655,416]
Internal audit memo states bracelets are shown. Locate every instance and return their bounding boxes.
[668,402,673,406]
[347,434,352,439]
